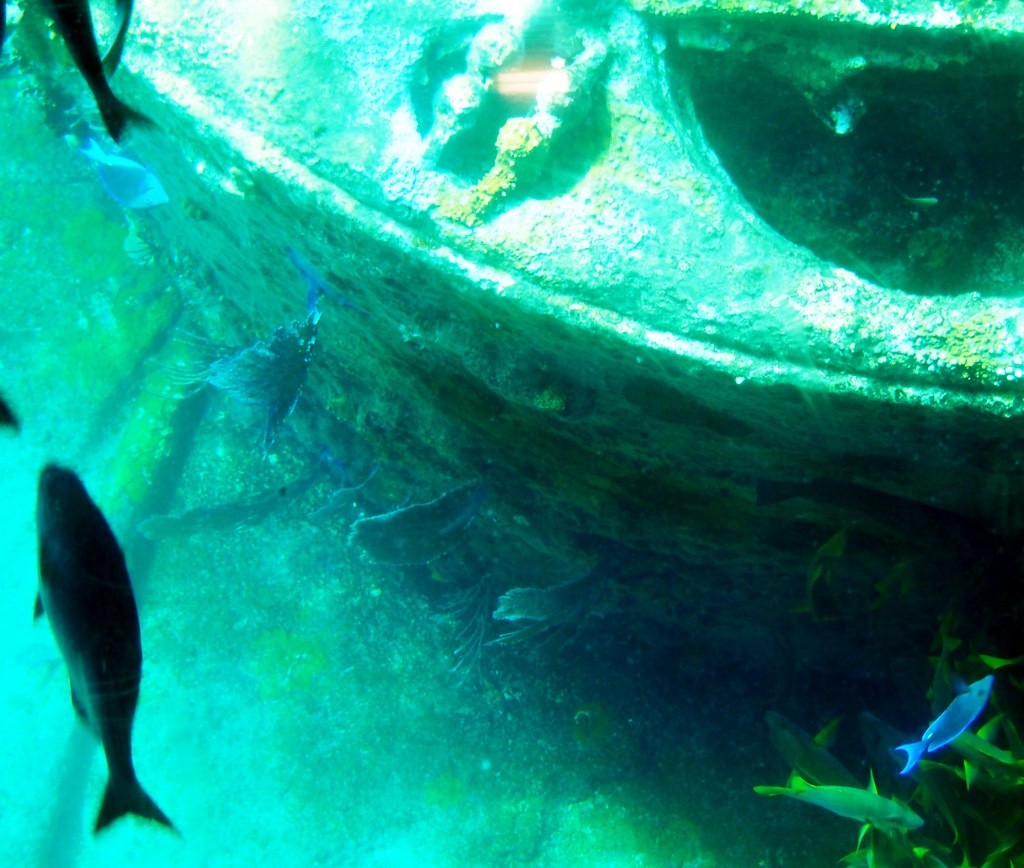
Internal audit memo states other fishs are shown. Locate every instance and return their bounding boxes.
[757,649,1023,867]
[2,0,683,840]
